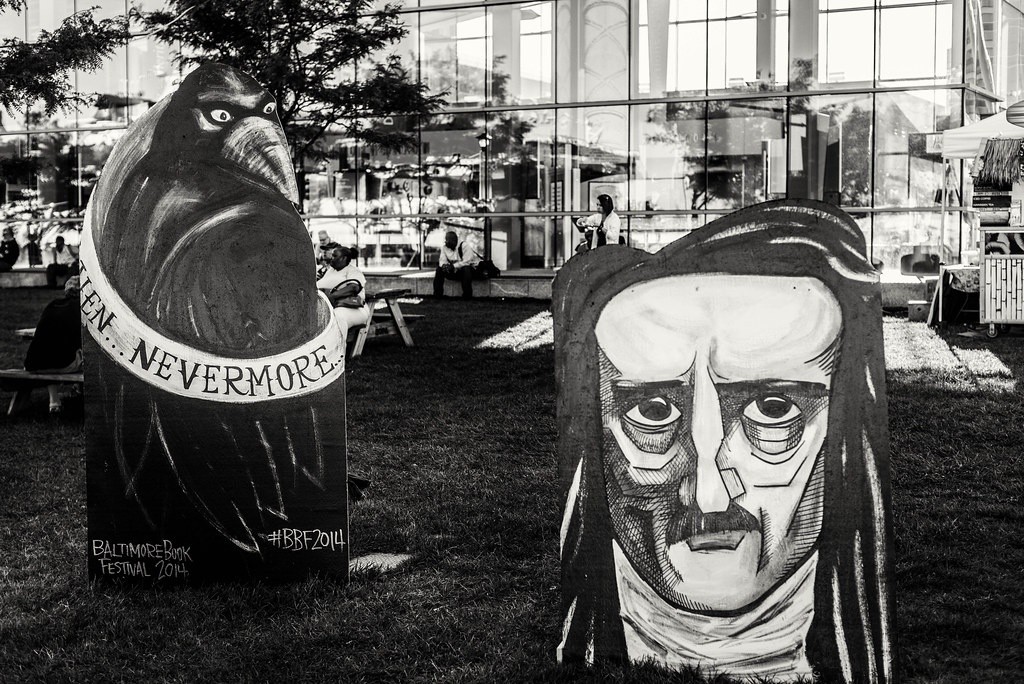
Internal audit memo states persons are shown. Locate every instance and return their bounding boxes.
[576,194,621,250]
[0,221,81,412]
[313,230,370,357]
[433,231,476,299]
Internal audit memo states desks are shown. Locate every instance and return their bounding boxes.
[352,288,414,358]
[7,328,38,415]
[939,265,982,323]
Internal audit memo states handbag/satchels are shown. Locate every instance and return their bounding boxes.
[476,259,501,280]
[320,278,363,308]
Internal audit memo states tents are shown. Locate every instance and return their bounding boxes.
[939,99,1024,322]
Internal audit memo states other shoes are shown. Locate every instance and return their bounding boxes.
[48,400,63,415]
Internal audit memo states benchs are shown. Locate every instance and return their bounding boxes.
[0,368,84,385]
